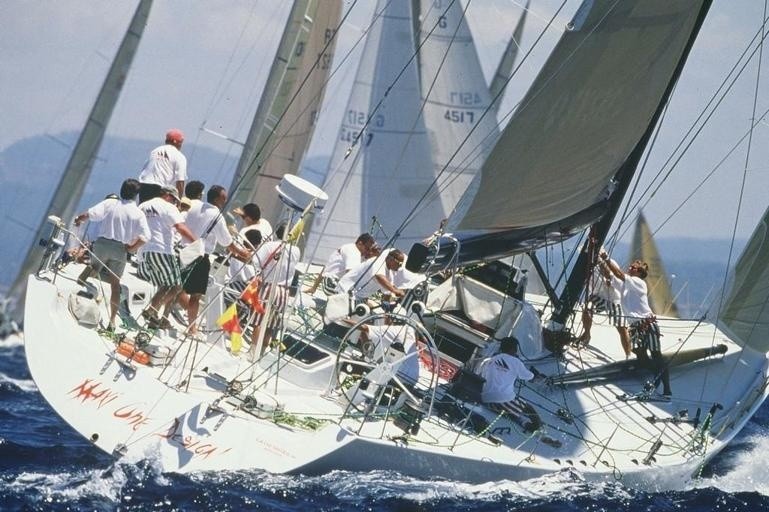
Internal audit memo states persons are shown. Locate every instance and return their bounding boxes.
[139,130,188,205]
[138,185,196,330]
[322,233,374,295]
[594,253,672,396]
[365,242,428,308]
[338,250,405,311]
[341,306,419,390]
[75,179,152,331]
[179,181,252,343]
[574,242,632,361]
[185,182,300,357]
[474,336,562,448]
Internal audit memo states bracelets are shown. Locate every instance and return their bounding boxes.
[604,256,609,261]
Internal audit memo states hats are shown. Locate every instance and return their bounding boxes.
[166,129,183,144]
[160,185,181,203]
[178,196,191,209]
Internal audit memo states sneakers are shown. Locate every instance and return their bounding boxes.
[142,307,175,332]
[77,264,93,285]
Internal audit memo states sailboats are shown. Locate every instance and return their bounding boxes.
[24,0,769,491]
